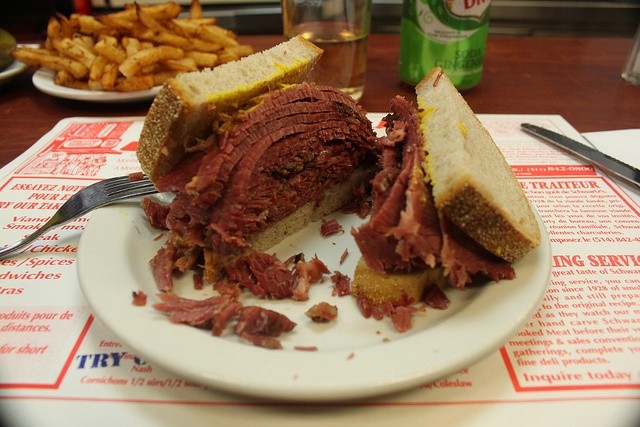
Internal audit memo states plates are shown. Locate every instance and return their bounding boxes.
[1,42,39,80]
[31,57,164,101]
[78,169,550,403]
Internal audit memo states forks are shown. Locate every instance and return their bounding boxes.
[0,170,158,276]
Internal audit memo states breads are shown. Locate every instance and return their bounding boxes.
[349,67,541,305]
[130,34,382,261]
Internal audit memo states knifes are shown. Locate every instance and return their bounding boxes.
[520,121,639,190]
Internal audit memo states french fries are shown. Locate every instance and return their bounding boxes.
[10,0,255,92]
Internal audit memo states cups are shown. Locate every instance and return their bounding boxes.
[282,1,372,105]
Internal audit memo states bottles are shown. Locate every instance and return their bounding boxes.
[398,2,489,91]
